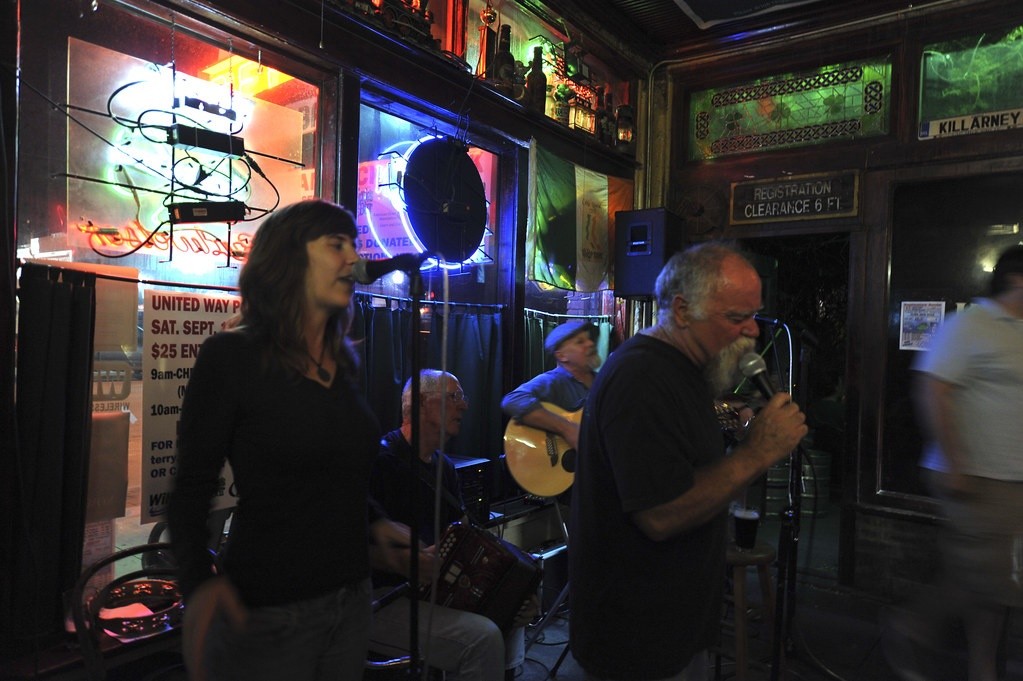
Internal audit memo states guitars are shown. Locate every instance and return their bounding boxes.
[504,396,587,499]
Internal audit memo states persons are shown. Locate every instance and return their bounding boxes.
[166,200,422,681]
[909,244,1023,681]
[567,242,808,681]
[501,320,603,450]
[365,369,539,681]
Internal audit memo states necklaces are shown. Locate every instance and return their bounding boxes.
[308,345,331,382]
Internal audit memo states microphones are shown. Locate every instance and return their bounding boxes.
[738,353,778,402]
[351,252,416,284]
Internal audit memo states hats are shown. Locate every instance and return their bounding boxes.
[544,321,589,353]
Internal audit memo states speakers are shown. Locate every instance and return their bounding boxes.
[612,208,680,302]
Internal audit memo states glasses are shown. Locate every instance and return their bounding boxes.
[423,390,468,401]
